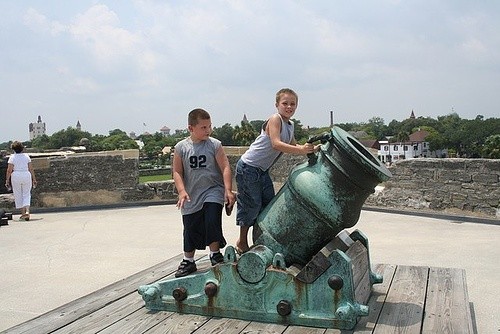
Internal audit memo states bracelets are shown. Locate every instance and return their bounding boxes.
[6,180,9,183]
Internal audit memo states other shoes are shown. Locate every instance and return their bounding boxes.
[21,214,29,221]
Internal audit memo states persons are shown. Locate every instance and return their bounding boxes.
[171,108,236,278]
[4,140,38,221]
[233,87,315,252]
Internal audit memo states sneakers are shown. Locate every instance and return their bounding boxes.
[175,259,197,277]
[210,252,224,266]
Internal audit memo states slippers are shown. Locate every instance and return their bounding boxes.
[234,245,250,256]
